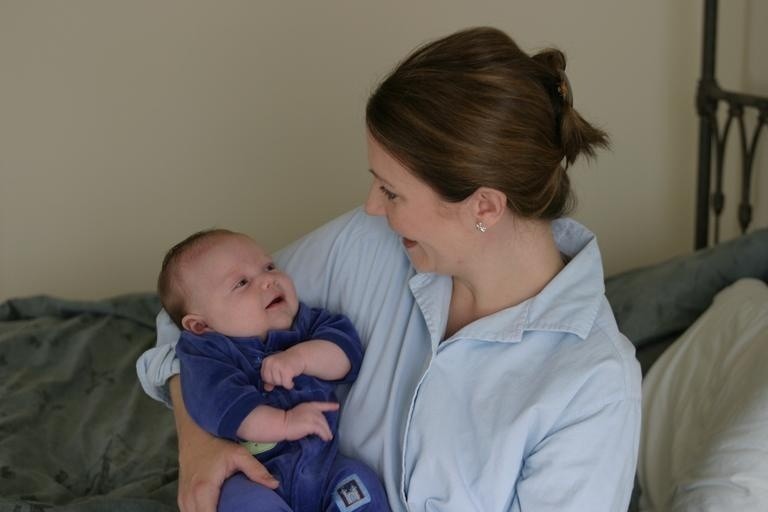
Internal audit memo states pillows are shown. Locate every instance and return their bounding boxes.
[638,277,768,512]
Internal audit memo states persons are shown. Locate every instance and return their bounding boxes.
[136,27,644,512]
[156,228,391,512]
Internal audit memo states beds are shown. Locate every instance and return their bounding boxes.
[0,2,768,512]
